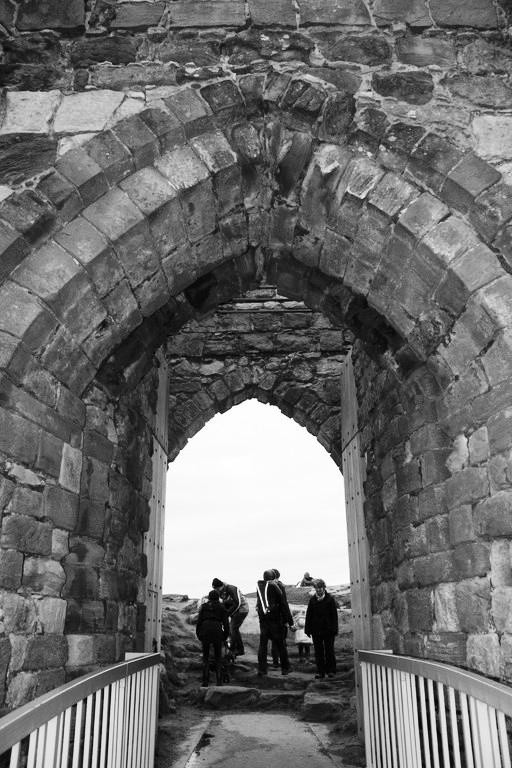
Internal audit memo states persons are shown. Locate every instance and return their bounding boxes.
[304,578,340,680]
[197,589,230,688]
[268,568,288,669]
[291,606,314,666]
[212,578,250,657]
[255,570,295,676]
[297,572,316,588]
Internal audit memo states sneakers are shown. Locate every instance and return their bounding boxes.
[201,669,334,687]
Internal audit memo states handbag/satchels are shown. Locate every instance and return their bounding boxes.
[265,607,279,619]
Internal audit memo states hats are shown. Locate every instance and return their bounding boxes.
[208,590,220,601]
[264,570,276,581]
[212,578,224,587]
